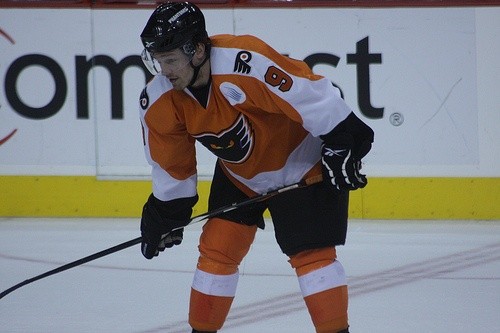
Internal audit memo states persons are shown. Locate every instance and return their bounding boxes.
[139,1,376,332]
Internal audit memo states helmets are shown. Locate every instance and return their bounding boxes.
[140,0,208,77]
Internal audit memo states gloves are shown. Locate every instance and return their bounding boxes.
[321,126,377,193]
[139,194,199,261]
